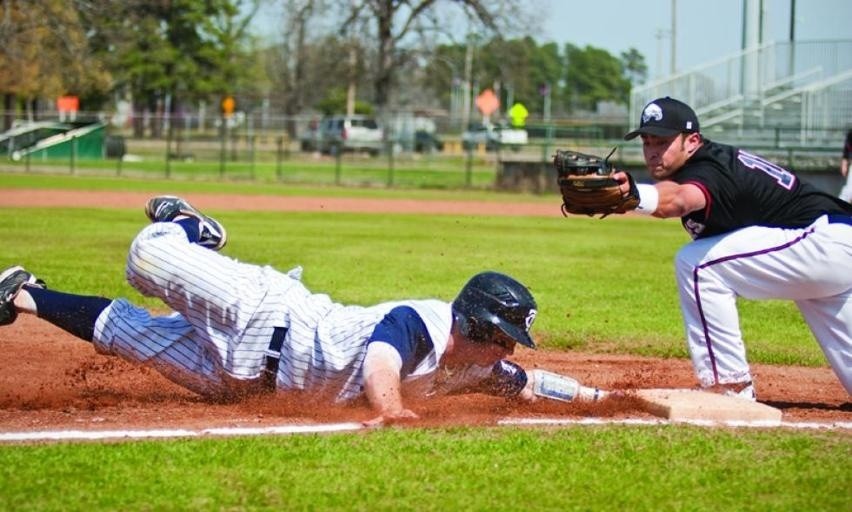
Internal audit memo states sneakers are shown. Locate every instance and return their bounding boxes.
[701,382,757,403]
[143,194,227,251]
[0,265,47,326]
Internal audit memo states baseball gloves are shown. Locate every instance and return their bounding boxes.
[554,146,640,219]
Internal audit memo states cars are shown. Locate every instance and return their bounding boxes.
[298,112,386,160]
[459,122,530,154]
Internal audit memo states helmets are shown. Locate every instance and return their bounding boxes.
[450,268,541,353]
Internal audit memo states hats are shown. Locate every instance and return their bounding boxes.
[624,95,702,145]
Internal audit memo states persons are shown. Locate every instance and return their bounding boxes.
[836,128,851,206]
[549,94,851,407]
[1,193,628,428]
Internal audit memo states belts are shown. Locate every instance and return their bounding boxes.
[261,325,289,397]
[826,212,852,225]
